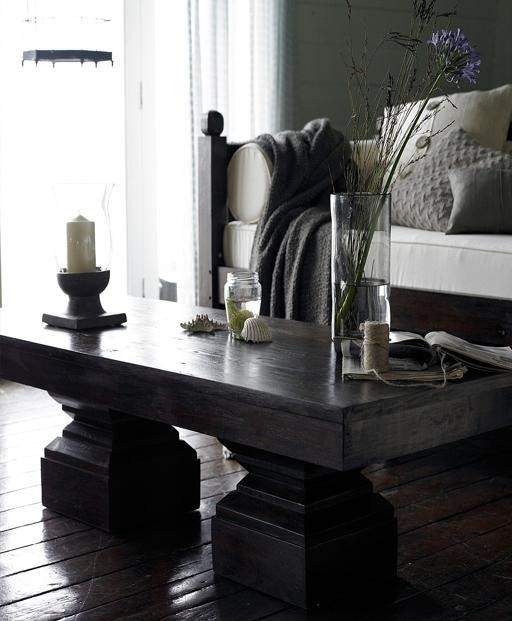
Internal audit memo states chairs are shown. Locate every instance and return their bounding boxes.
[197,82,510,348]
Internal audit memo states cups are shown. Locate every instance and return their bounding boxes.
[225,271,262,339]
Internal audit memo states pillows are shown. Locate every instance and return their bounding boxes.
[372,84,510,233]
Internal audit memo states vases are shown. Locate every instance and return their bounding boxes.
[325,190,396,347]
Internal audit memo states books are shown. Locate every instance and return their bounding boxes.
[386,331,511,376]
[341,340,467,381]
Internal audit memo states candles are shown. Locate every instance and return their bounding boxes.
[63,215,100,273]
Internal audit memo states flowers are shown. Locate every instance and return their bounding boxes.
[327,2,484,342]
